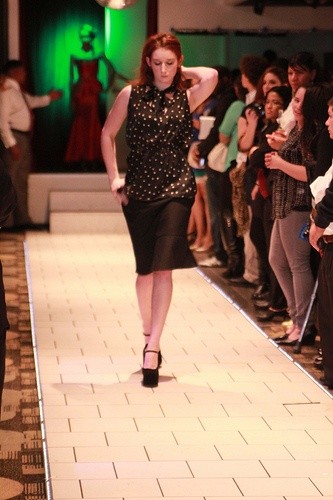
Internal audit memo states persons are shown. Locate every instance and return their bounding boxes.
[0,60,64,231]
[102,32,218,387]
[60,24,117,172]
[186,48,333,390]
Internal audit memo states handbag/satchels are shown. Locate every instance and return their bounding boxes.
[206,141,229,173]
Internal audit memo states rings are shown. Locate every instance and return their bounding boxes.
[118,188,122,193]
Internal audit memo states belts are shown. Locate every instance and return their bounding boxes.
[11,129,31,136]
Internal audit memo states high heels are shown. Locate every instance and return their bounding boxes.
[272,325,318,345]
[142,343,163,386]
[189,241,215,253]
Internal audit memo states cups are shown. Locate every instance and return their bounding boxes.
[197,116,216,140]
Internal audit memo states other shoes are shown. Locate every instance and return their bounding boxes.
[227,276,257,288]
[222,269,243,279]
[256,309,288,322]
[197,256,226,268]
[13,220,44,231]
[252,282,275,300]
[254,297,286,310]
